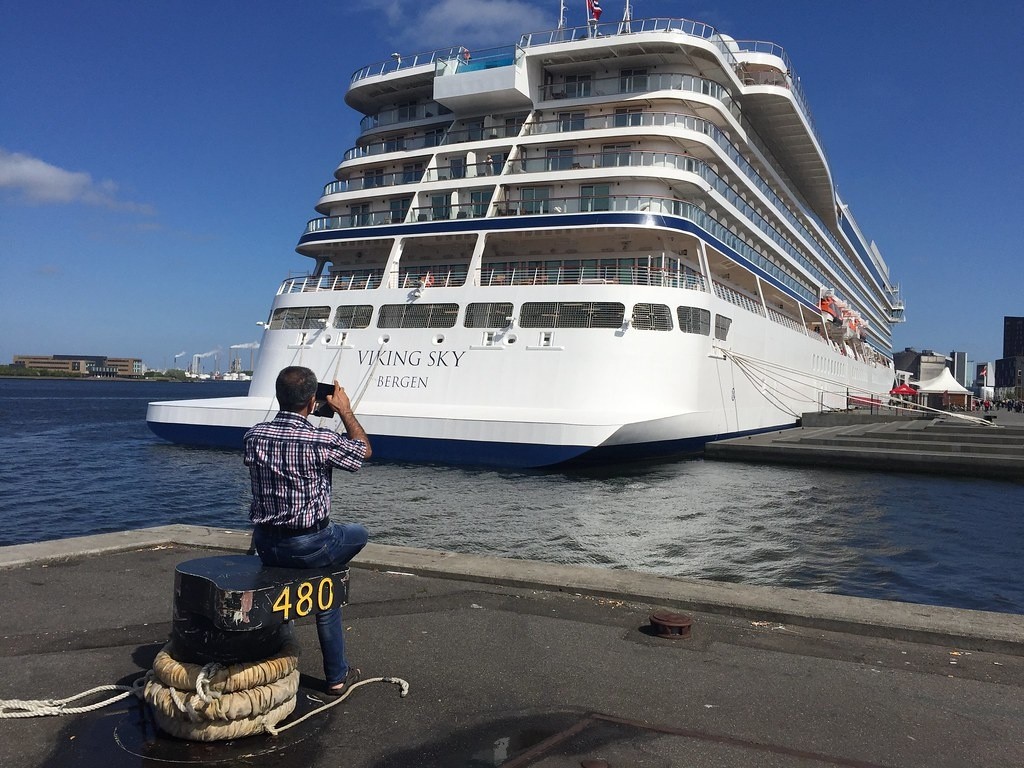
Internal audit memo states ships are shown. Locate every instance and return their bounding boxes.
[146,2,905,449]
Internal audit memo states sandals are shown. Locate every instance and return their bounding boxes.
[329,666,363,696]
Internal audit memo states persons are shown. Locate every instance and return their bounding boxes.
[242,365,375,697]
[482,155,494,176]
[975,394,1022,413]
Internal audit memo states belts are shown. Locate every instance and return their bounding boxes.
[259,518,330,537]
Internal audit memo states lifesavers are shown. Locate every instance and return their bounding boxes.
[421,275,434,287]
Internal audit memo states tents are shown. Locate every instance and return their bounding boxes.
[909,366,975,412]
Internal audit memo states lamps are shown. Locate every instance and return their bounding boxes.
[256,322,270,330]
[623,318,634,330]
[506,316,517,326]
[318,319,329,329]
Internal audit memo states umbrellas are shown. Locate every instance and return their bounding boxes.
[890,382,917,395]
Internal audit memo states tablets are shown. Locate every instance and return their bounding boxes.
[311,383,336,418]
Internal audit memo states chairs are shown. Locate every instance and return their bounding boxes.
[493,274,508,285]
[534,275,550,284]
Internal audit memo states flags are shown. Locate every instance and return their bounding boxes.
[588,0,603,21]
